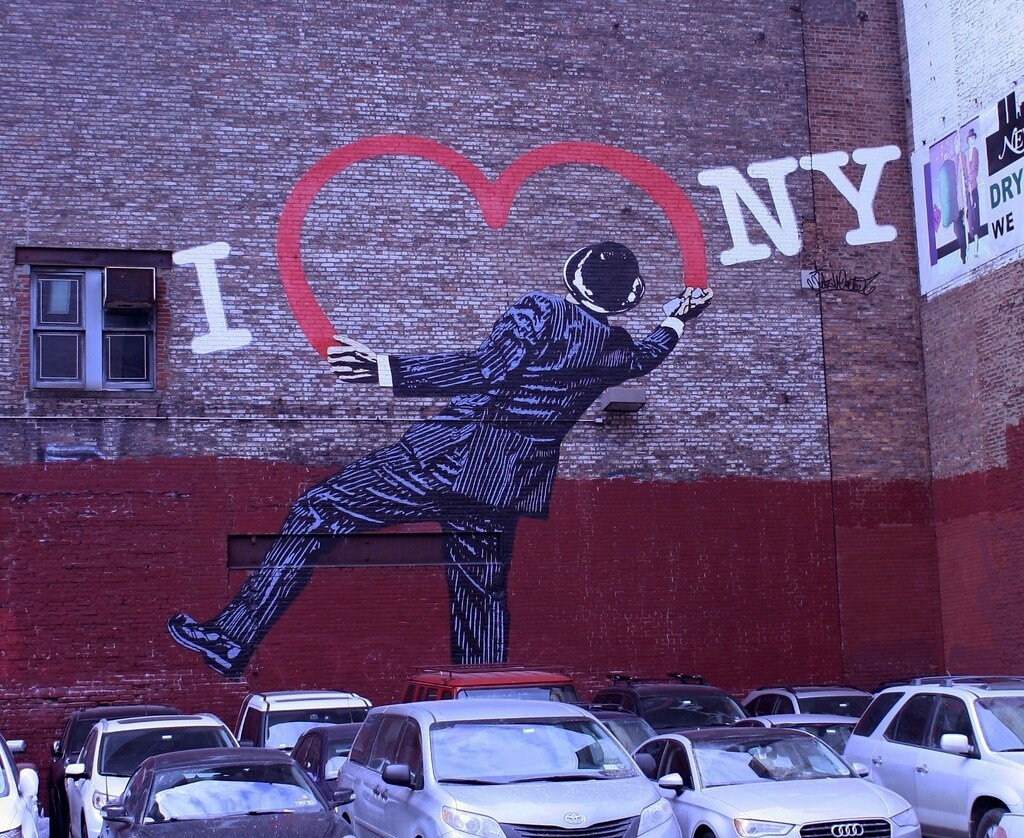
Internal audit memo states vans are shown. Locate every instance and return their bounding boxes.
[399,661,584,704]
[331,697,683,838]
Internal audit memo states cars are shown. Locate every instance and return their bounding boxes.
[565,702,659,761]
[0,732,51,838]
[96,745,358,838]
[287,720,367,804]
[626,726,921,838]
[728,712,861,757]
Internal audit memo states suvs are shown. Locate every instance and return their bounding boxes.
[63,710,242,838]
[46,703,184,837]
[741,680,876,719]
[590,670,752,735]
[232,689,374,757]
[841,673,1024,838]
